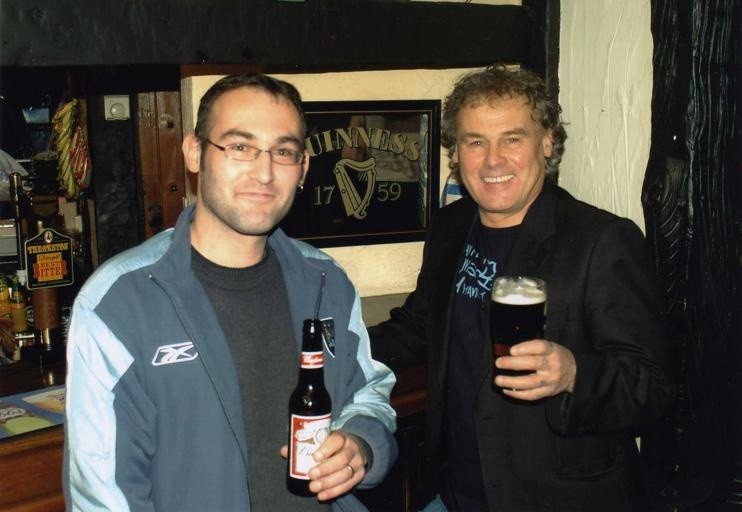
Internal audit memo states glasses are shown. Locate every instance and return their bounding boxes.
[195,134,308,165]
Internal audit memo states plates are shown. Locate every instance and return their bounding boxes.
[35,149,58,161]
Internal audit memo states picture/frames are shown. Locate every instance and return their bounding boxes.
[279,99,446,249]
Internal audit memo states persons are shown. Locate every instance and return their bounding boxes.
[62,74,400,512]
[341,114,422,234]
[366,61,673,512]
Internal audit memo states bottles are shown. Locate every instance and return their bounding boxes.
[286,318,333,496]
[10,274,29,333]
[0,275,13,320]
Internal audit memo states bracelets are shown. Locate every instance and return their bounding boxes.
[346,464,355,478]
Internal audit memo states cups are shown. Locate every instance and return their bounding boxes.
[489,274,547,379]
